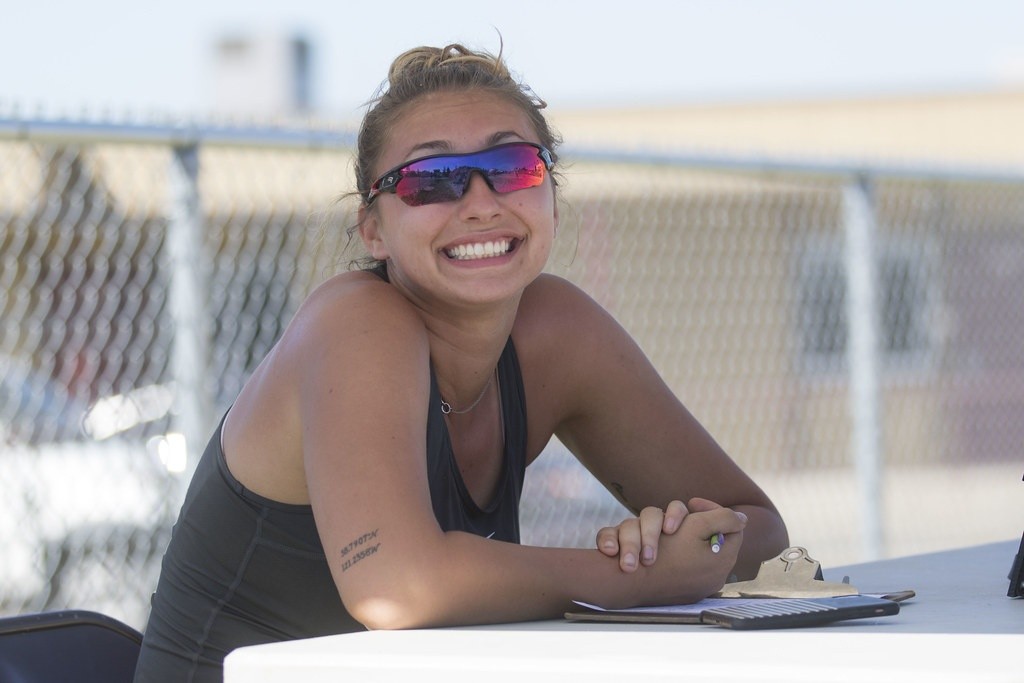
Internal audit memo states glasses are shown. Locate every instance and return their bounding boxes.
[366,142,554,209]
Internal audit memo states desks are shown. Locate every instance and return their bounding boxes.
[222,535,1023,683]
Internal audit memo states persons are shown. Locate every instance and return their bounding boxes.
[130,42,791,683]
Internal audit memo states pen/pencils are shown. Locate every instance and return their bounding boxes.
[710,532,724,554]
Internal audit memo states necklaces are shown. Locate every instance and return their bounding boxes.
[439,368,497,414]
